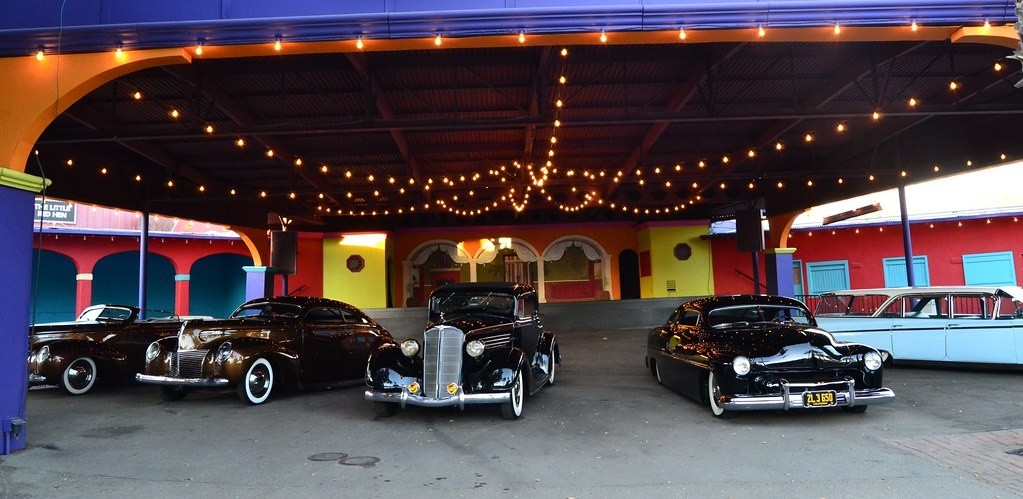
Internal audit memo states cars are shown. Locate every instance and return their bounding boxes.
[26,304,217,398]
[131,296,402,407]
[645,293,896,419]
[794,283,1023,369]
[362,282,563,421]
